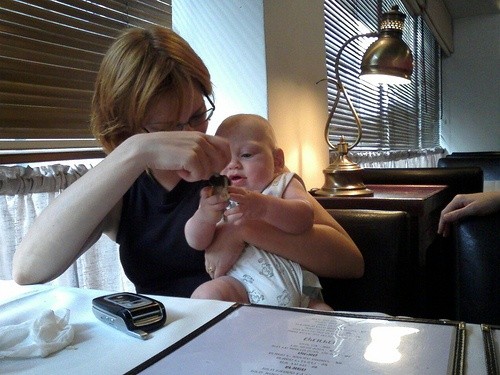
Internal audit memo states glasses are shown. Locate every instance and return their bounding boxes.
[137,92,215,133]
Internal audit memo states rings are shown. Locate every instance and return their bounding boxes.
[207,266,216,273]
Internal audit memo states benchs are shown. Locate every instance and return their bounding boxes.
[361,151,500,204]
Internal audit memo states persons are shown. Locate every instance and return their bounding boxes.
[184,113,334,311]
[12,25,365,299]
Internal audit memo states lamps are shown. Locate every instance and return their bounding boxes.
[313,4,413,198]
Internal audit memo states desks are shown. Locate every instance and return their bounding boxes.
[308,184,448,319]
[0,281,488,375]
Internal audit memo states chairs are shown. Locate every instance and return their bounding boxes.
[319,209,409,318]
[455,215,499,328]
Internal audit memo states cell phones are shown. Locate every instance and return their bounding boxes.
[92,291,167,340]
